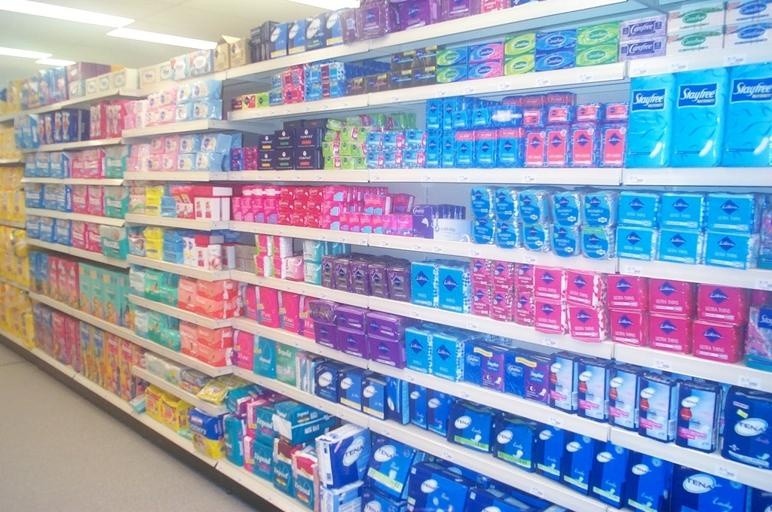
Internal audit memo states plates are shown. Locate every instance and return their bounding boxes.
[0,1,768,512]
